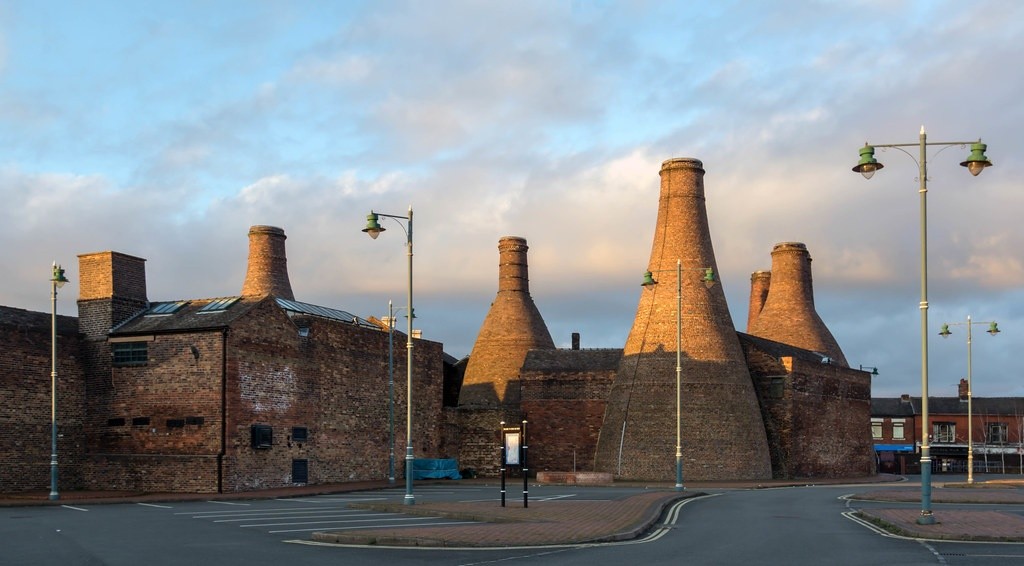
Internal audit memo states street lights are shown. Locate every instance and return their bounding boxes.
[850,125,997,528]
[640,257,716,489]
[47,261,68,502]
[938,315,1001,485]
[361,205,417,505]
